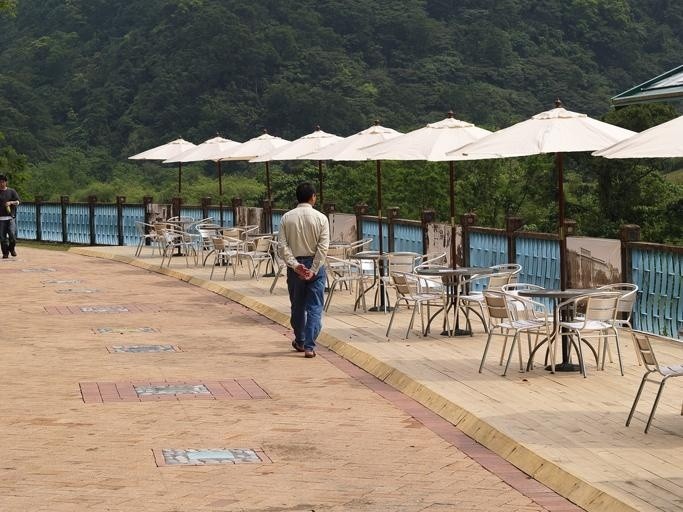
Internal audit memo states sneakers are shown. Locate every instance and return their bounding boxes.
[10,250,17,256]
[3,254,8,258]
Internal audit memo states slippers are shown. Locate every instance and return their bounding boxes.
[292,340,315,358]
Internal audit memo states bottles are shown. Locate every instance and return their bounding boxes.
[6,204,11,215]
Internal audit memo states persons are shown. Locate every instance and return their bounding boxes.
[0,175,20,258]
[277,182,330,358]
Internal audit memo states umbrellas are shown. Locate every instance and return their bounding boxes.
[162,132,243,262]
[296,120,406,254]
[248,124,345,210]
[210,128,293,272]
[591,115,683,162]
[127,135,198,255]
[443,98,638,289]
[333,109,494,270]
